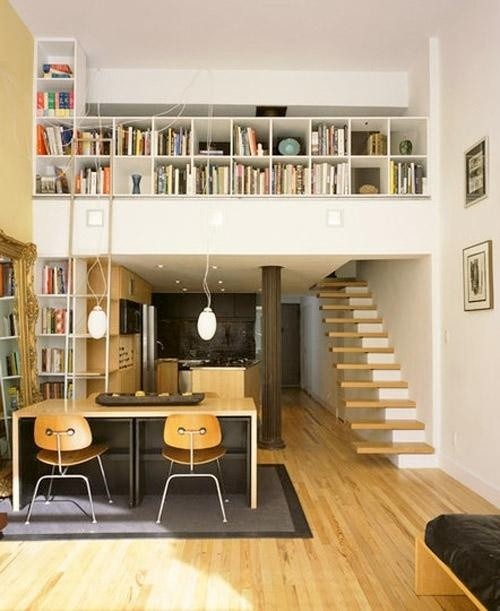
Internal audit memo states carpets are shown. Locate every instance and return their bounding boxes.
[0,455,313,540]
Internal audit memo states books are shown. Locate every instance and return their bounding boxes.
[195,125,311,195]
[37,63,73,193]
[0,261,74,410]
[310,123,350,196]
[366,133,427,195]
[75,125,193,194]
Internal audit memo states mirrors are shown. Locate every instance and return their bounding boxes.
[0,230,43,498]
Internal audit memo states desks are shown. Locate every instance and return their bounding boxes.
[12,398,258,512]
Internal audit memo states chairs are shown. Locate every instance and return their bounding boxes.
[25,415,113,525]
[156,413,229,524]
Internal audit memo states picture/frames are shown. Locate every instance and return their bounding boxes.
[463,135,487,208]
[463,240,493,311]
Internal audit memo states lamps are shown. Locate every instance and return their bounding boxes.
[197,47,217,342]
[77,64,109,339]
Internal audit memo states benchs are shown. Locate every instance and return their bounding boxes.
[414,514,500,611]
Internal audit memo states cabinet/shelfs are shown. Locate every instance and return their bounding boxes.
[37,255,117,399]
[0,260,20,460]
[119,267,153,306]
[36,38,430,196]
